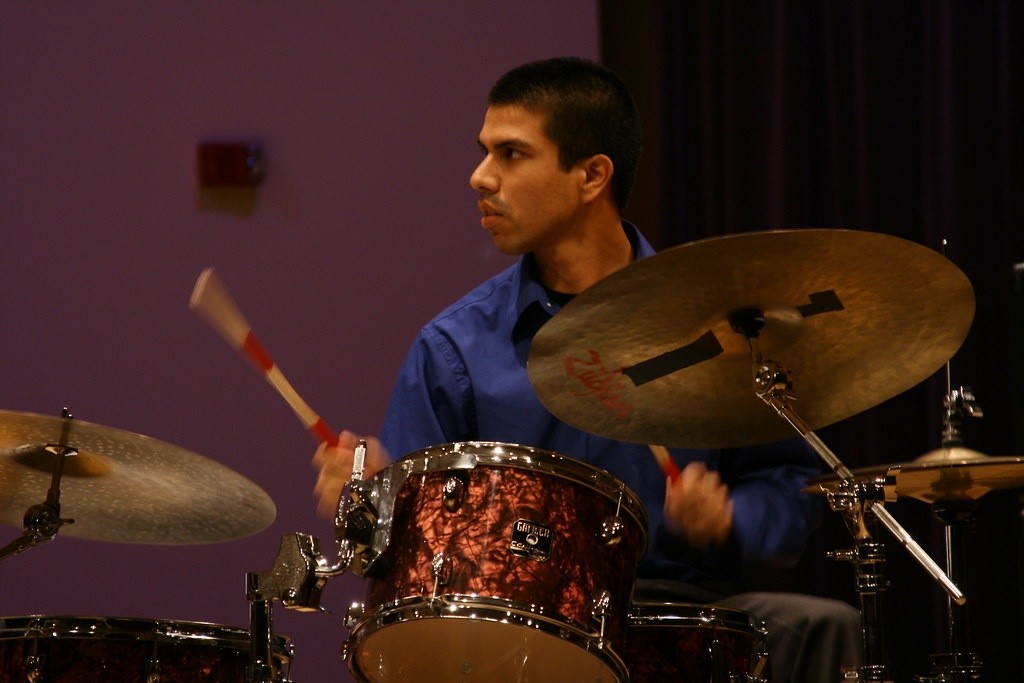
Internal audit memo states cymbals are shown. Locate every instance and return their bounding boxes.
[798,446,1024,504]
[529,224,980,450]
[0,408,279,549]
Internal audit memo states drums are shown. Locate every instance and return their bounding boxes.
[342,439,654,683]
[623,598,769,683]
[1,614,296,683]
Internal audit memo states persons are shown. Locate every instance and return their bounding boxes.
[313,55,865,683]
